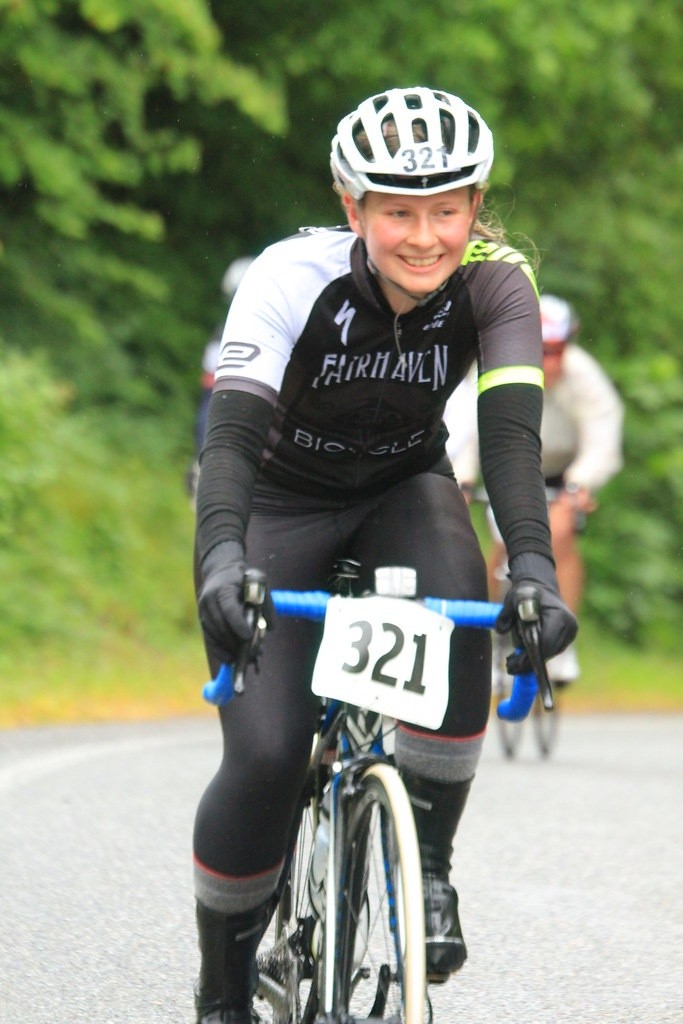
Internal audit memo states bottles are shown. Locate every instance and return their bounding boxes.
[308,791,374,917]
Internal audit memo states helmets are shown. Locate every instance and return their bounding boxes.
[329,86,495,201]
[539,295,572,346]
[221,257,255,295]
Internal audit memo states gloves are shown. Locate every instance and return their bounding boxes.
[495,573,579,675]
[197,541,276,665]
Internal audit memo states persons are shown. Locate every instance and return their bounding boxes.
[200,259,625,692]
[193,86,578,1022]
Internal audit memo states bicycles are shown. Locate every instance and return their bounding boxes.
[458,478,589,761]
[200,551,555,1023]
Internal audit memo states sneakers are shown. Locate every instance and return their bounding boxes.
[193,960,262,1024]
[419,843,467,974]
